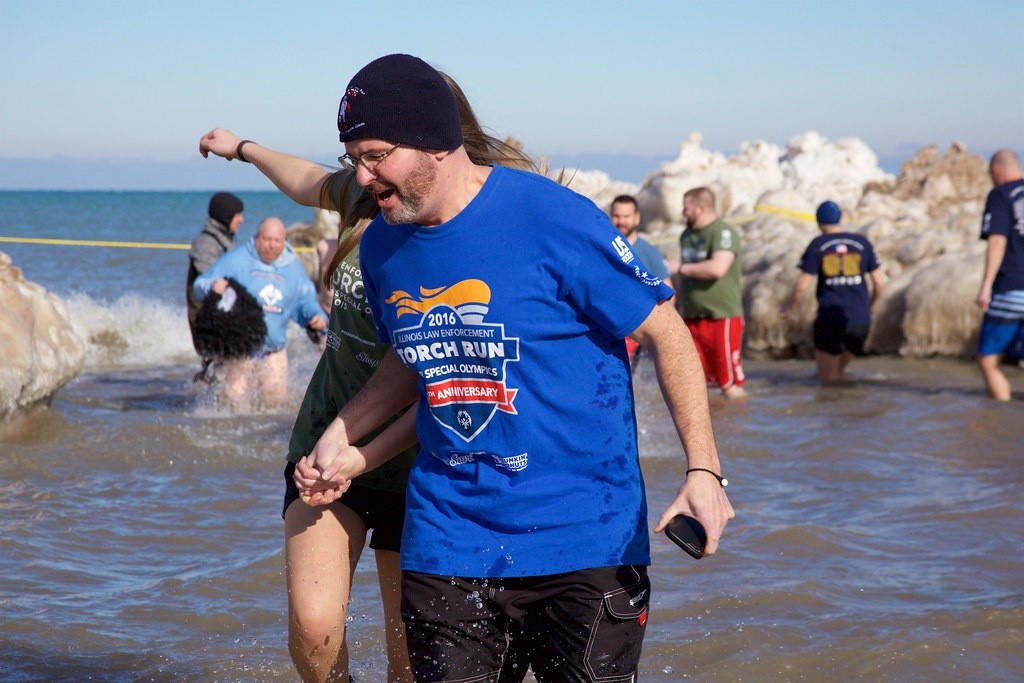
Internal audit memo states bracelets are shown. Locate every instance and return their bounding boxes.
[237,141,257,163]
[685,469,729,488]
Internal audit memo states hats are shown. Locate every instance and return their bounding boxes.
[817,201,842,225]
[336,54,462,151]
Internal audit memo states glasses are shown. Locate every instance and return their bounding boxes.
[338,143,403,170]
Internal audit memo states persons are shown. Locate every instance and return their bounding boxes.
[969,145,1024,403]
[202,70,538,681]
[790,198,883,383]
[190,215,327,413]
[294,48,735,683]
[609,195,675,379]
[666,187,749,399]
[183,191,247,389]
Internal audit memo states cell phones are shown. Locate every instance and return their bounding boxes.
[664,507,708,560]
